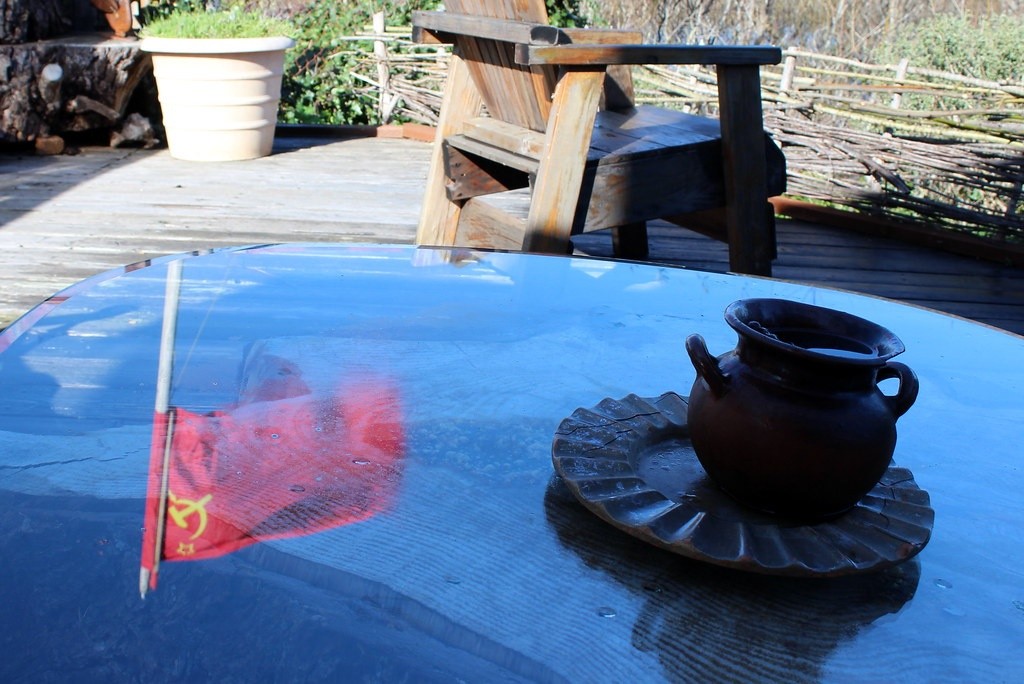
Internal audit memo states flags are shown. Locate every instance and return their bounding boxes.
[138,373,409,587]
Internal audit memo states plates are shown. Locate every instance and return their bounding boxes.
[551,390,936,576]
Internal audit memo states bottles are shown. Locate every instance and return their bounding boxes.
[685,297,920,523]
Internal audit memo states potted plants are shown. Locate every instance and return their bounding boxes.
[134,8,302,163]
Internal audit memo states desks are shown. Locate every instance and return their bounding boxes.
[0,242,1024,684]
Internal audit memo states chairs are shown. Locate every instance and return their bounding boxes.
[411,0,788,280]
